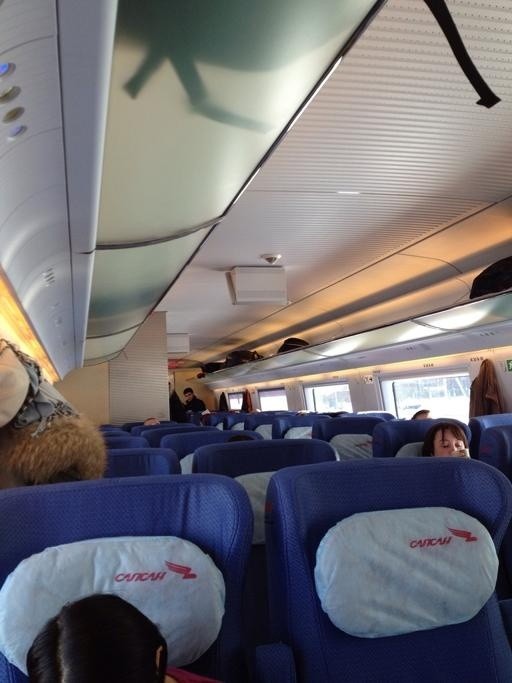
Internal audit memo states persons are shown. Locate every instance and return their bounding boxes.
[182,387,207,411]
[421,420,470,457]
[144,417,160,425]
[26,595,218,682]
[411,409,432,420]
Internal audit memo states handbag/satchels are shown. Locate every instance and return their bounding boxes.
[200,350,264,373]
[278,338,309,354]
[470,256,512,299]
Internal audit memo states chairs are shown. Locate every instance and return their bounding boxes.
[1,405,511,683]
